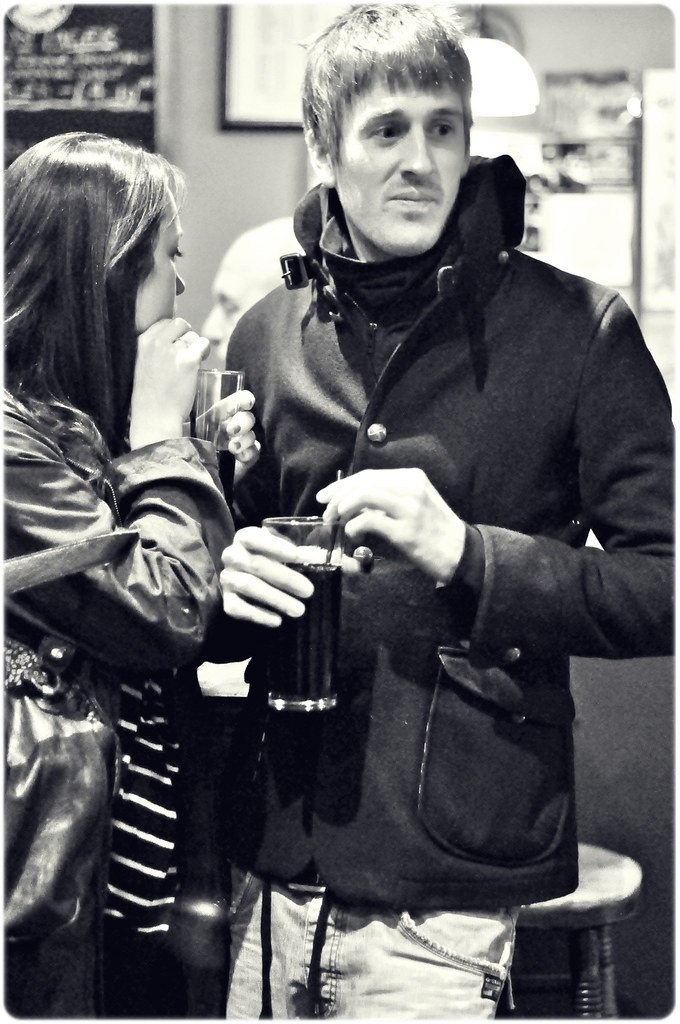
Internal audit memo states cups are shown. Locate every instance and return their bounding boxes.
[264,517,338,709]
[195,368,244,508]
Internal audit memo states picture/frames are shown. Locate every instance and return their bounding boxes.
[218,0,350,132]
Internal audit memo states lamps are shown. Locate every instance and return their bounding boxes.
[455,39,545,175]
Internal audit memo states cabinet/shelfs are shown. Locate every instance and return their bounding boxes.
[513,67,642,285]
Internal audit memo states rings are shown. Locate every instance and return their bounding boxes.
[176,336,190,349]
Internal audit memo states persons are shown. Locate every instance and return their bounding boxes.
[216,2,675,1020]
[4,131,262,1019]
[202,215,308,366]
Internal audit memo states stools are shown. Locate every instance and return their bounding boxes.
[515,842,643,1019]
